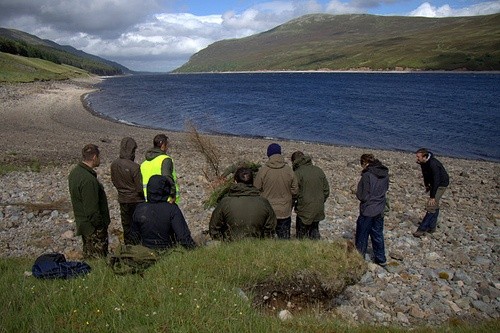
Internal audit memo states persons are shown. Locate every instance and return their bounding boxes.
[254,143,298,240]
[350,154,389,265]
[209,165,278,243]
[111,137,145,246]
[68,143,111,258]
[140,134,179,206]
[413,148,449,237]
[132,175,198,253]
[291,150,330,239]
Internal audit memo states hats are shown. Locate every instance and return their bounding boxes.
[267,143,281,157]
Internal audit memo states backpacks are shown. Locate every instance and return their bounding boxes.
[33,254,92,279]
[105,244,158,275]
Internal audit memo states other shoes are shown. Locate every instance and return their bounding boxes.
[413,229,426,237]
[427,228,436,233]
[371,257,386,266]
